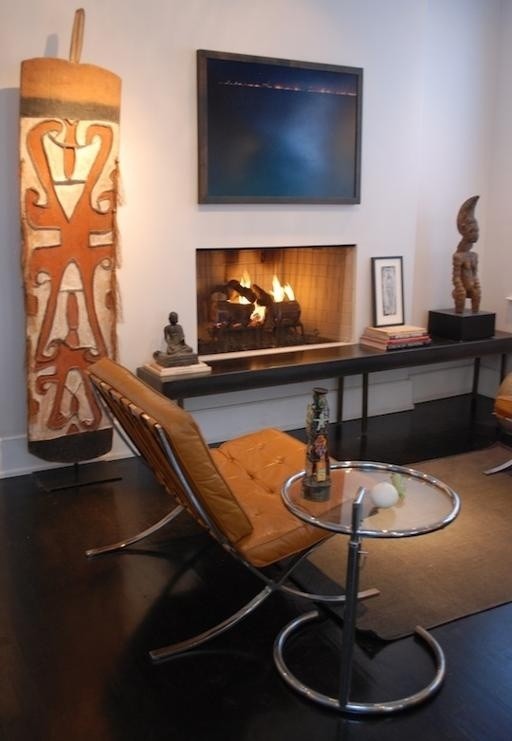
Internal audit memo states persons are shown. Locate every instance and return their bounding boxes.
[450,195,482,313]
[164,311,193,355]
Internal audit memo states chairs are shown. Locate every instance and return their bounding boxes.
[481,370,512,477]
[83,356,382,662]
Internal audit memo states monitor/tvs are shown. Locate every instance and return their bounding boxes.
[195,47,364,207]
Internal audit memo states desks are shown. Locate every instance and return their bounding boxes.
[273,460,462,716]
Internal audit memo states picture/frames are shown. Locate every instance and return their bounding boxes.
[371,256,405,328]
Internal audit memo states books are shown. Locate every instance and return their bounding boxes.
[359,327,432,353]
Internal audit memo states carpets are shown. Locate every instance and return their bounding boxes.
[279,442,512,642]
